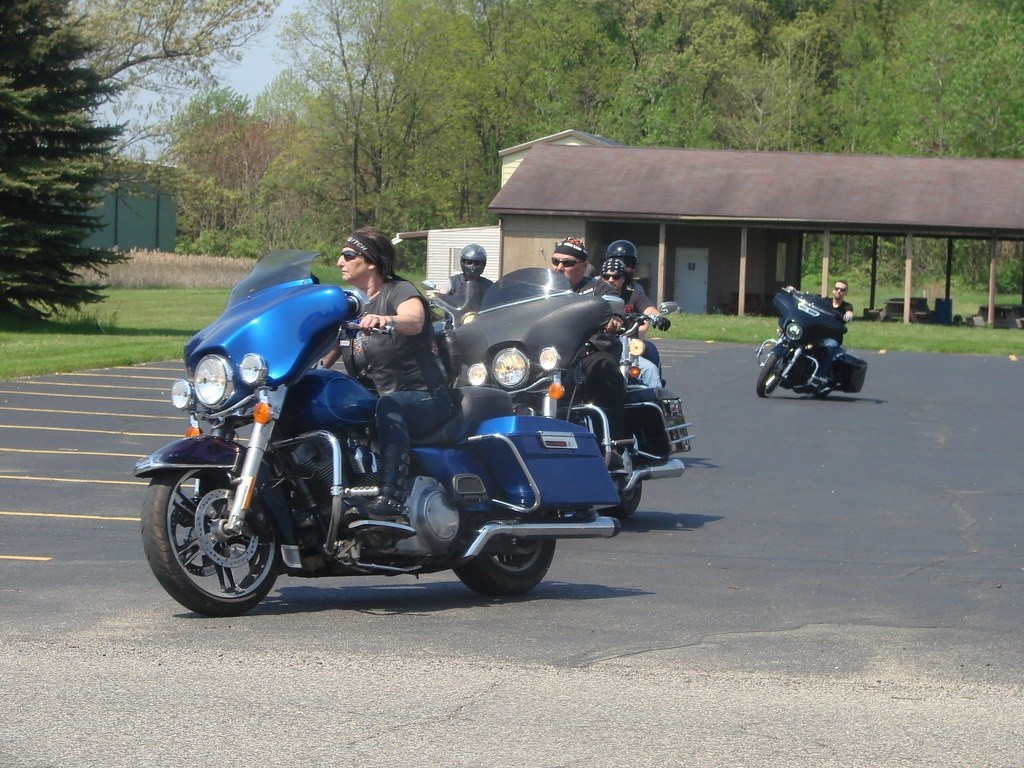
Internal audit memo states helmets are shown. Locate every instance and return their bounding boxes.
[460,244,486,276]
[606,241,636,269]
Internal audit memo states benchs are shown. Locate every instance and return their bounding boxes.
[870,310,926,324]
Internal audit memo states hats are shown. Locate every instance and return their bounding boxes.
[602,257,625,276]
[555,236,588,264]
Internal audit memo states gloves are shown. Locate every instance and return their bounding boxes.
[652,316,671,333]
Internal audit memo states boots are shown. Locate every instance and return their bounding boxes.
[343,443,412,527]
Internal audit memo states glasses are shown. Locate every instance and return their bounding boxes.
[601,273,621,280]
[465,259,481,266]
[551,257,583,267]
[342,251,362,260]
[834,285,847,292]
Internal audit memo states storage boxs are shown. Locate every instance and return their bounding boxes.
[615,386,672,468]
[829,352,868,393]
[463,413,622,510]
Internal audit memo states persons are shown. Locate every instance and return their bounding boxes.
[427,244,494,333]
[605,241,668,385]
[787,280,854,393]
[600,257,672,387]
[551,235,627,469]
[313,228,452,521]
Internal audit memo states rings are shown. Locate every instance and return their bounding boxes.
[371,316,374,319]
[375,317,379,319]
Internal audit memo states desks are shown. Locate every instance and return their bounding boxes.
[879,300,918,324]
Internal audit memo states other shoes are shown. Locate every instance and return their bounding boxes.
[604,452,626,480]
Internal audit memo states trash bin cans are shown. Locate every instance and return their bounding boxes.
[934,297,953,326]
[625,277,648,301]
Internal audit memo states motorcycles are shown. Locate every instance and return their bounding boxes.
[424,267,695,520]
[751,287,868,400]
[132,250,620,618]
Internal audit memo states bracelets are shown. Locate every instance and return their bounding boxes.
[389,316,394,325]
